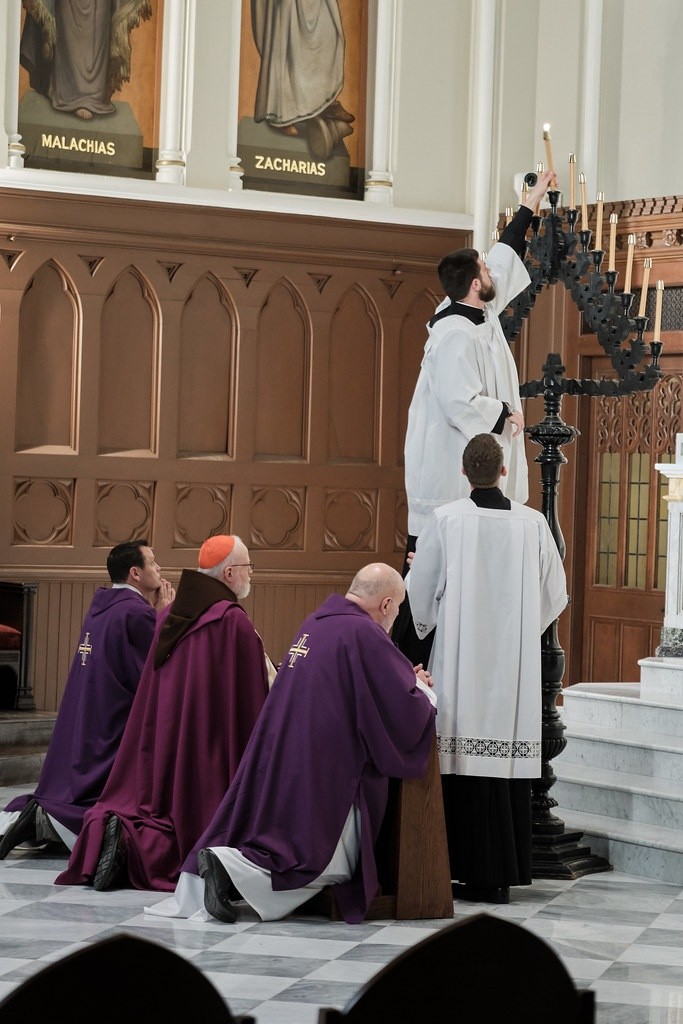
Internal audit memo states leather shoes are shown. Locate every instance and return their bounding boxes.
[450,880,508,905]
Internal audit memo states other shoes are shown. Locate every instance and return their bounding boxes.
[198,848,237,923]
[92,815,127,891]
[0,800,39,862]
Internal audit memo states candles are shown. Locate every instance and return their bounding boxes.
[654,281,664,342]
[506,206,513,226]
[480,251,487,261]
[544,131,556,187]
[579,172,588,230]
[609,213,618,271]
[638,259,652,317]
[624,234,636,294]
[492,231,499,244]
[521,183,528,202]
[596,191,604,249]
[533,161,543,215]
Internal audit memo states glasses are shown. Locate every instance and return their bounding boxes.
[225,561,254,574]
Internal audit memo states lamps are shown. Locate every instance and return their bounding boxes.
[568,153,576,209]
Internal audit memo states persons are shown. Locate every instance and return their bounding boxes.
[399,432,568,903]
[390,169,559,671]
[54,535,280,891]
[0,539,177,860]
[143,563,438,924]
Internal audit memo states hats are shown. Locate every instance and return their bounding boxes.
[198,535,235,569]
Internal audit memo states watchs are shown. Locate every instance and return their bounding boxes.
[508,406,515,416]
